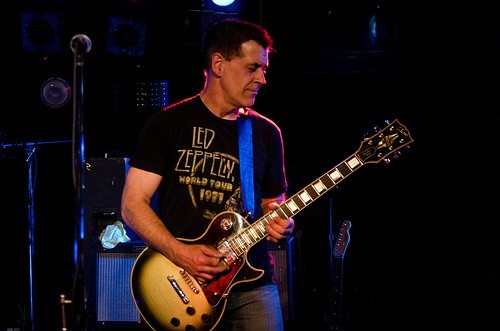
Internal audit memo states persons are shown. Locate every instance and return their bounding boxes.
[121,17,294,331]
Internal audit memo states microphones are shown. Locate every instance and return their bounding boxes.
[70,34,92,53]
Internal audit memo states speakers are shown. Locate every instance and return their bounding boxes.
[269,236,295,325]
[94,240,147,324]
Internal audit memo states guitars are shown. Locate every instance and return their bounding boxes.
[129,118,415,331]
